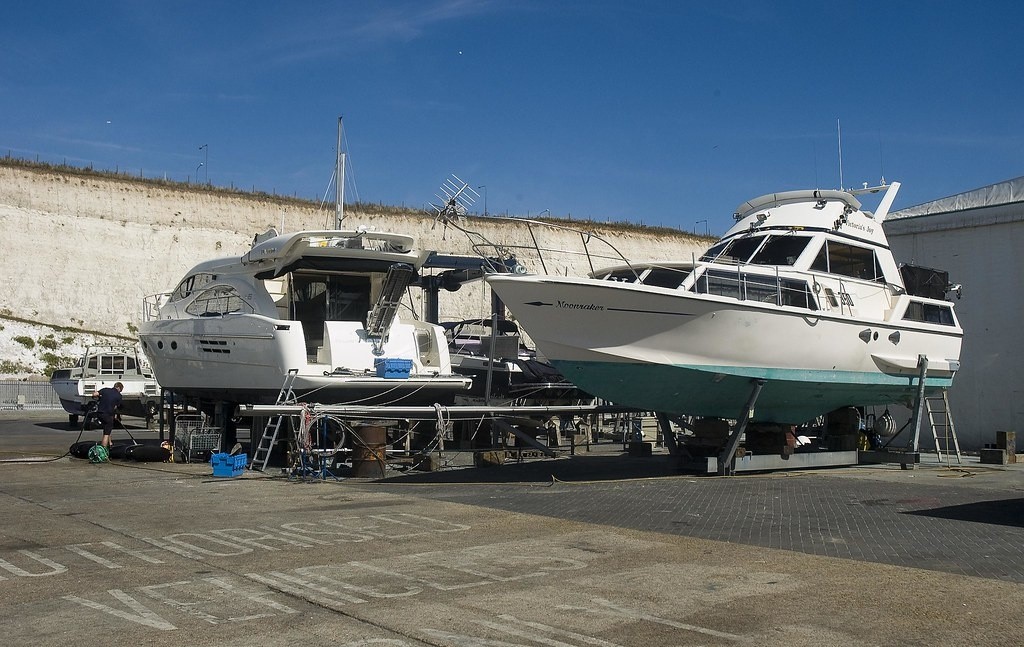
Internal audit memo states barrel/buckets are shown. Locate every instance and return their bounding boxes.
[351,426,387,479]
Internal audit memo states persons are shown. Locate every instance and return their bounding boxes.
[92,382,124,449]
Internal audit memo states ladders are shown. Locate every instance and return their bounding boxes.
[250,368,303,470]
[923,389,962,464]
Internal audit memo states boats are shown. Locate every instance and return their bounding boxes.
[427,115,964,425]
[134,114,600,422]
[48,343,162,417]
